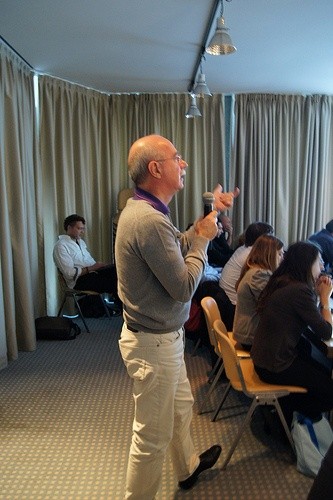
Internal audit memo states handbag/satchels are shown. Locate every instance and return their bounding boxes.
[291,410,333,478]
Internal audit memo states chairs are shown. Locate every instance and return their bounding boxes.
[192,280,308,471]
[56,268,112,333]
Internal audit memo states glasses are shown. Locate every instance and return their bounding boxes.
[157,154,182,162]
[319,259,325,265]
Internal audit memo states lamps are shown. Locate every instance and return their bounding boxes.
[185,82,201,119]
[206,0,237,56]
[192,58,212,98]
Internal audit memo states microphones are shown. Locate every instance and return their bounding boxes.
[202,191,215,251]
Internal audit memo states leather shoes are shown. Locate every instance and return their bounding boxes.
[179,444,222,490]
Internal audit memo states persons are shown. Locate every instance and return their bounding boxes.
[114,134,240,500]
[189,217,333,462]
[307,441,333,500]
[53,214,123,316]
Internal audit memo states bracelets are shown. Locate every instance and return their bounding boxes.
[320,305,332,311]
[85,267,89,274]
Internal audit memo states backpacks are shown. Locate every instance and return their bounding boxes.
[34,315,80,341]
